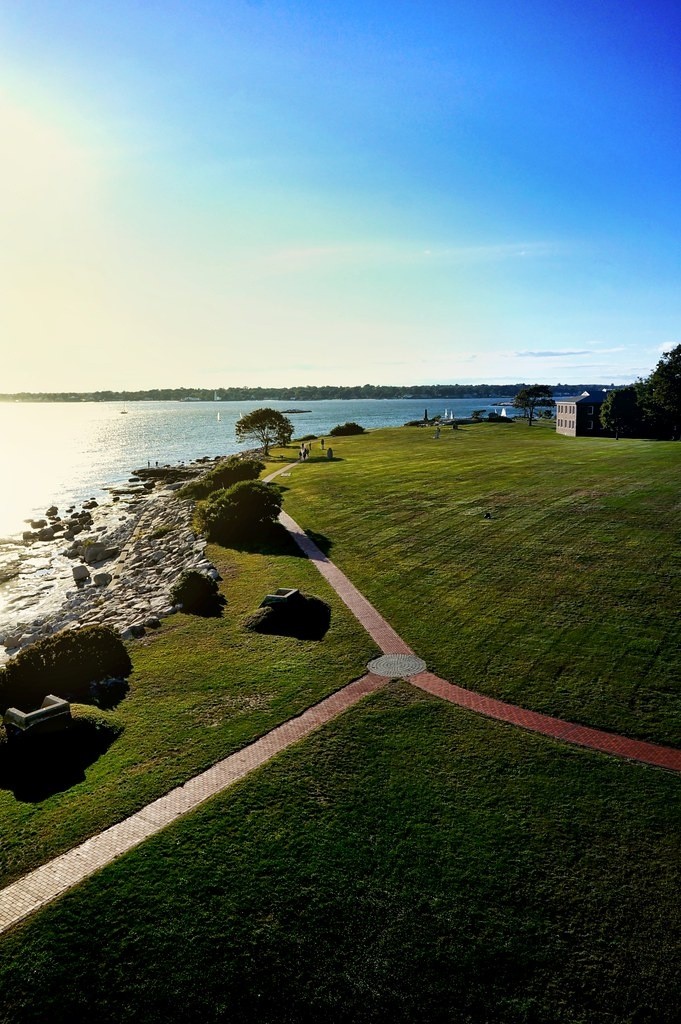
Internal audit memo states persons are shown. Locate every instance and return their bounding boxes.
[436,426,440,438]
[321,439,324,450]
[299,441,311,461]
